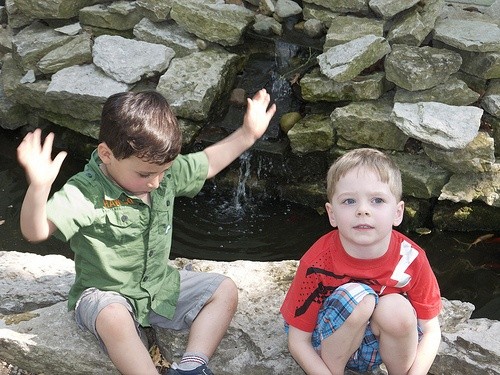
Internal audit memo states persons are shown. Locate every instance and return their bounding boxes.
[280,148,442,375]
[16,89,277,374]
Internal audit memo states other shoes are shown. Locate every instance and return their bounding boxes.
[167,362,214,375]
